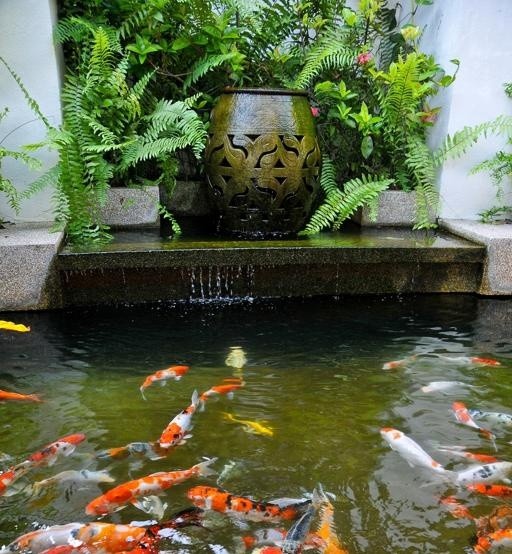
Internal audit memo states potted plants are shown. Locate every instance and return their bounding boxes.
[1,23,203,245]
[189,0,325,240]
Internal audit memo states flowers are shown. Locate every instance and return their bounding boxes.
[299,46,507,238]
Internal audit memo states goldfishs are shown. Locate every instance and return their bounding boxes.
[0,430,346,554]
